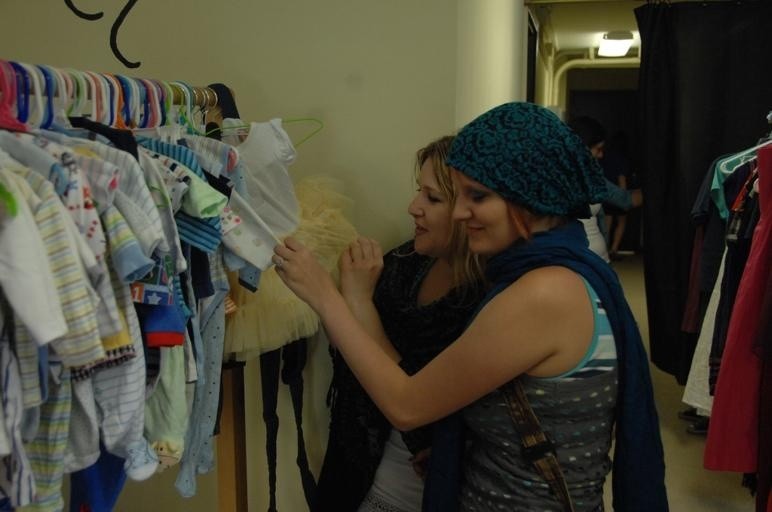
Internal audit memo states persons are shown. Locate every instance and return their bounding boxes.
[602,166,630,262]
[312,128,490,509]
[584,128,644,244]
[270,101,672,509]
[567,116,642,268]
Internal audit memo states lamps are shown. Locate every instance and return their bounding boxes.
[595,28,634,60]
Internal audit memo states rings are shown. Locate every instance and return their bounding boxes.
[275,257,285,267]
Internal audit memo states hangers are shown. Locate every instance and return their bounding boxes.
[709,110,772,176]
